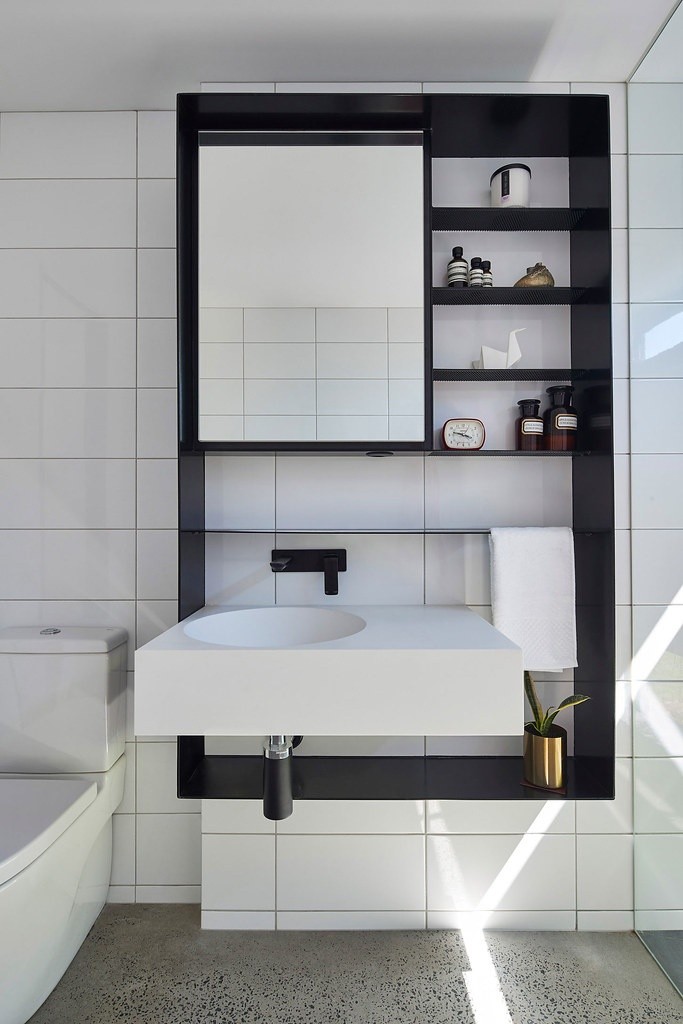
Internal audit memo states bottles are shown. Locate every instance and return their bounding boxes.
[468,257,484,287]
[516,398,544,451]
[447,246,469,288]
[482,261,493,288]
[543,385,579,451]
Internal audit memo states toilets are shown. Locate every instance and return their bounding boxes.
[1,627,129,1024]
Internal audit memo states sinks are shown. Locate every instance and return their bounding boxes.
[183,606,367,645]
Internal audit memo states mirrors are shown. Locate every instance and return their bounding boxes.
[196,137,427,450]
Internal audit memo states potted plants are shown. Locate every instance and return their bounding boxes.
[525,671,592,789]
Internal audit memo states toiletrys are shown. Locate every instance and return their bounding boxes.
[516,384,577,450]
[447,245,493,288]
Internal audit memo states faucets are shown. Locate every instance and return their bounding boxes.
[270,558,293,572]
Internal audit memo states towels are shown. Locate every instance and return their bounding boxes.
[485,528,579,673]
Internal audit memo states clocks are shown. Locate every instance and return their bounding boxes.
[440,418,485,451]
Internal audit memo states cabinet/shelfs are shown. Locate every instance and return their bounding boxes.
[423,93,611,456]
